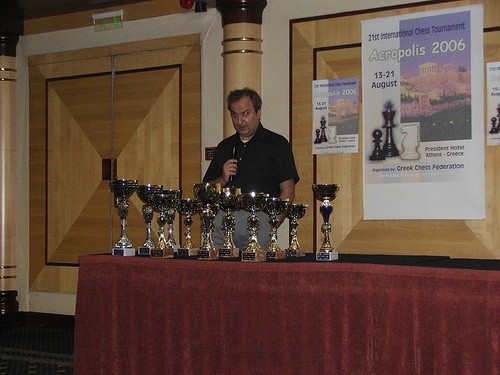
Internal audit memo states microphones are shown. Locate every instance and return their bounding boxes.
[229,141,237,182]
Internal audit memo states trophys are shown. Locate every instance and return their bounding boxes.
[311,183,341,262]
[109,179,308,260]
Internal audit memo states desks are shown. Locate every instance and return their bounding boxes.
[72,250,500,375]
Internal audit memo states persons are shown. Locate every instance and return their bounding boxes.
[202,86,300,251]
